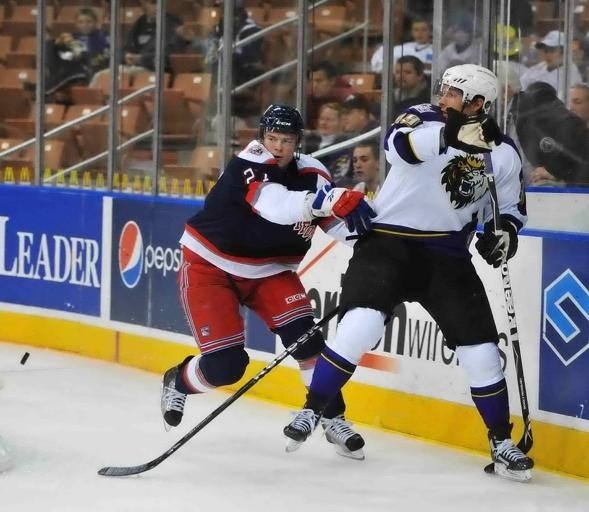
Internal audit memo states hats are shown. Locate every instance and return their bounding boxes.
[340,92,370,112]
[492,23,523,58]
[533,29,565,50]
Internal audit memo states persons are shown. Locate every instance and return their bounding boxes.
[160,103,366,451]
[21,1,589,194]
[283,63,534,471]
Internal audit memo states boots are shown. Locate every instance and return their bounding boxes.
[283,392,322,442]
[319,412,365,454]
[487,423,534,472]
[159,355,196,427]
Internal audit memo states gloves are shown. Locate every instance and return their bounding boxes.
[474,211,525,269]
[311,180,378,238]
[443,104,503,155]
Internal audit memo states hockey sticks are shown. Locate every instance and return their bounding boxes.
[484,153,534,473]
[98,310,339,475]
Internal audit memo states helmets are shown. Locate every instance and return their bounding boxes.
[432,61,499,119]
[258,103,305,163]
